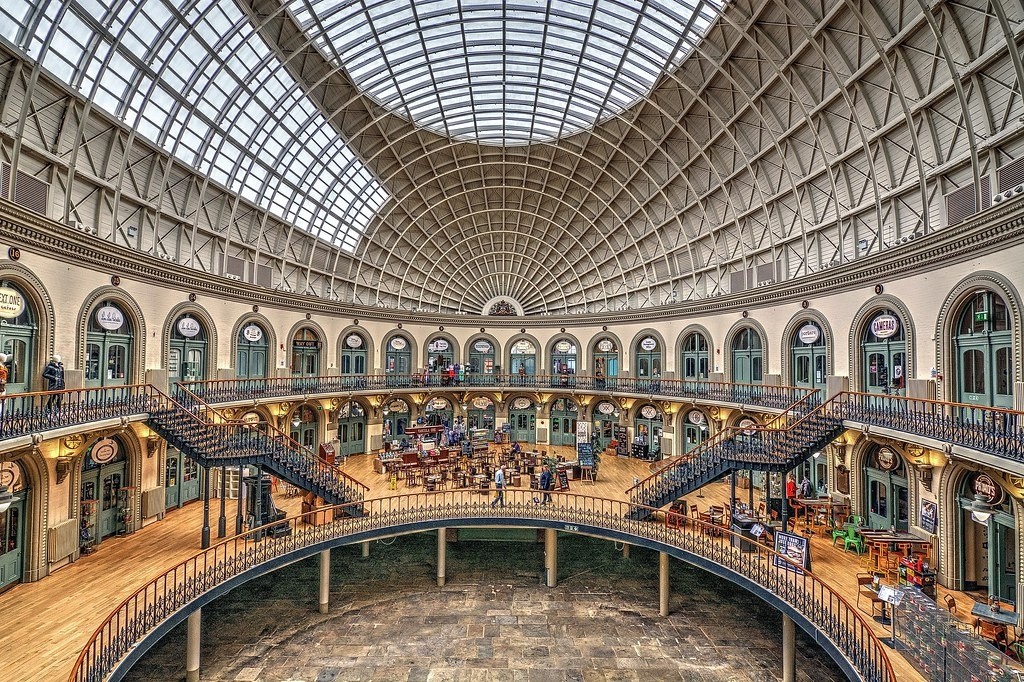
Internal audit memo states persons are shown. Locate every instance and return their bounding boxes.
[453,420,465,446]
[42,355,65,418]
[490,465,507,508]
[511,441,520,456]
[787,474,811,499]
[399,438,409,451]
[519,363,525,383]
[449,363,464,380]
[540,465,552,505]
[0,353,8,422]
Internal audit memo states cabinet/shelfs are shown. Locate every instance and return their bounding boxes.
[891,585,1024,682]
[218,468,249,500]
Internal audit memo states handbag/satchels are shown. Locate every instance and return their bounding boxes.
[494,491,498,498]
[539,485,546,490]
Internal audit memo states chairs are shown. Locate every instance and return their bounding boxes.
[384,445,565,490]
[856,572,881,617]
[944,593,978,634]
[667,498,901,585]
[976,595,1015,642]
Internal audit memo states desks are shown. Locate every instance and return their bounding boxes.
[394,463,418,467]
[863,583,891,625]
[792,498,847,507]
[381,459,404,462]
[505,469,518,472]
[970,601,1020,627]
[860,532,930,545]
[470,474,488,477]
[424,476,441,479]
[426,458,448,462]
[524,451,542,455]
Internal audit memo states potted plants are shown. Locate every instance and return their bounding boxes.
[541,453,558,491]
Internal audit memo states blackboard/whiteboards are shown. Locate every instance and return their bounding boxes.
[554,470,570,489]
[578,442,594,467]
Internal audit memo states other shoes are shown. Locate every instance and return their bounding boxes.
[547,499,552,502]
